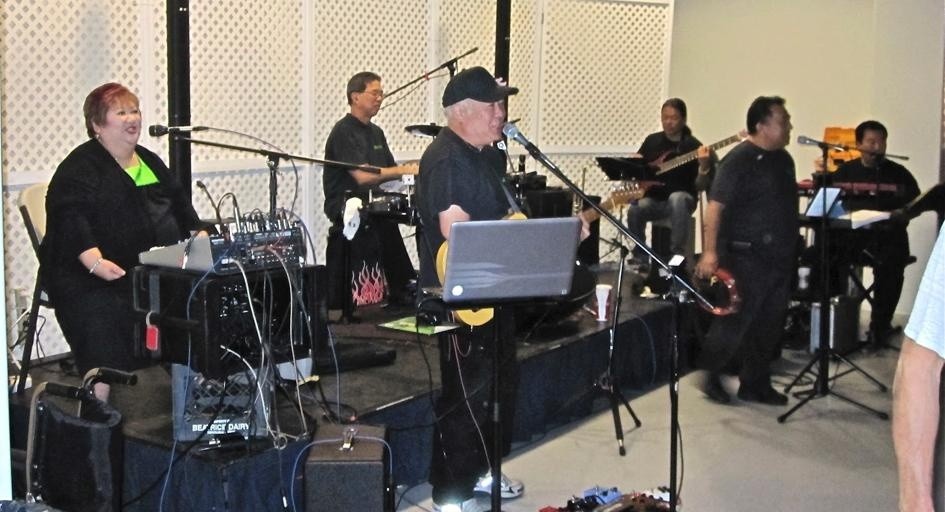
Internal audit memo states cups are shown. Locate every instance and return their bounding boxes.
[592,284,614,321]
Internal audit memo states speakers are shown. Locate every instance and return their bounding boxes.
[304,423,394,512]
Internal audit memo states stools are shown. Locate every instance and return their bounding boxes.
[320,224,394,330]
[843,249,918,339]
[648,215,697,274]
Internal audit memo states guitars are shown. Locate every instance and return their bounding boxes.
[618,129,753,200]
[435,185,644,327]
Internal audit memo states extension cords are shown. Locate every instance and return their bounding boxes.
[14,287,30,345]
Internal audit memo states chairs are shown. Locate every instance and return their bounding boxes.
[12,178,86,395]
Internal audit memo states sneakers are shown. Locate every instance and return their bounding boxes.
[430,498,487,512]
[736,378,788,406]
[472,463,523,499]
[693,372,730,404]
[441,66,519,107]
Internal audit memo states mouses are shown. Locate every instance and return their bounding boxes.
[417,310,440,326]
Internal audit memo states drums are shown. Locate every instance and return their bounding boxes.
[381,181,416,223]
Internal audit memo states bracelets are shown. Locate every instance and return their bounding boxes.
[88,257,106,274]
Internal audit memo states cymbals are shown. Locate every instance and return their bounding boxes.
[405,125,447,136]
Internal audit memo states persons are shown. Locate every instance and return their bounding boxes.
[36,83,210,403]
[417,67,591,512]
[886,222,945,512]
[696,97,800,404]
[625,99,721,275]
[810,121,920,347]
[323,73,419,319]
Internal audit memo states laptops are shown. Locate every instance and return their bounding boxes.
[422,217,582,304]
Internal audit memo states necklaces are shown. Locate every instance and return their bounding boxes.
[108,149,138,165]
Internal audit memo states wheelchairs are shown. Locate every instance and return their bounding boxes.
[8,366,138,511]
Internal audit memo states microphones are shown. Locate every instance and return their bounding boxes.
[149,125,208,136]
[197,181,226,237]
[798,136,842,149]
[503,123,562,175]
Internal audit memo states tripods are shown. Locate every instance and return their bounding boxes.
[258,152,318,427]
[777,151,888,423]
[546,245,641,455]
[784,220,887,394]
